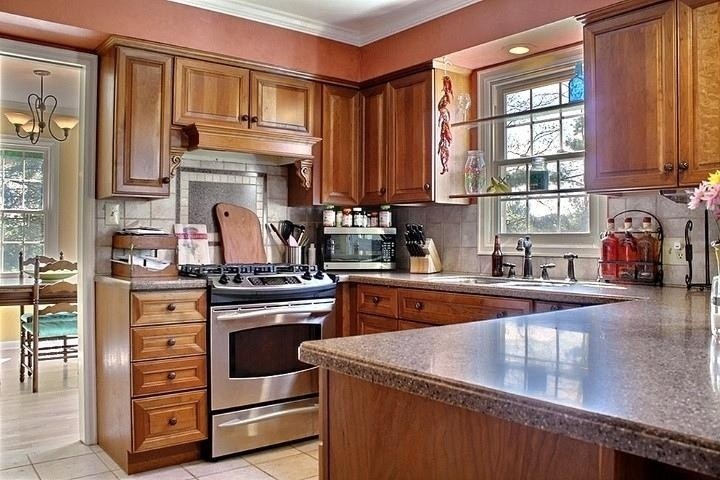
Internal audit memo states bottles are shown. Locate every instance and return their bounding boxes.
[568,61,584,103]
[637,217,659,282]
[456,93,471,121]
[491,235,503,277]
[308,244,316,266]
[464,150,487,194]
[601,219,619,280]
[322,204,393,226]
[618,217,637,281]
[530,157,548,192]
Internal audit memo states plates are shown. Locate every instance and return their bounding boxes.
[116,229,169,235]
[21,269,78,283]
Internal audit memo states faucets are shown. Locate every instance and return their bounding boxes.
[563,253,579,281]
[516,235,534,279]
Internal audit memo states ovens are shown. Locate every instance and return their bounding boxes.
[207,273,337,459]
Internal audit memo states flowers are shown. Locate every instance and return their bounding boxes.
[685,171,720,222]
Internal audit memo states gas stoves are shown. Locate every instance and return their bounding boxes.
[178,263,331,277]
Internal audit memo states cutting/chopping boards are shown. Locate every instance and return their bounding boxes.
[215,203,267,265]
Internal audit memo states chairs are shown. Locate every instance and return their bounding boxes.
[20,255,77,393]
[18,251,77,377]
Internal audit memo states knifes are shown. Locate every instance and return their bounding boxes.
[404,222,427,257]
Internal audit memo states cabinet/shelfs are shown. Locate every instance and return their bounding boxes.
[398,288,533,331]
[583,0,720,197]
[450,98,585,199]
[351,283,398,336]
[95,283,209,475]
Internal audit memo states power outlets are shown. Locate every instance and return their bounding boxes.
[674,242,686,261]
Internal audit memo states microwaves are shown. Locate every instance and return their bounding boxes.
[323,227,397,270]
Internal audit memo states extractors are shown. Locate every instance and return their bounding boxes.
[169,122,324,191]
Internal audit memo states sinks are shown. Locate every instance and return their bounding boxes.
[502,280,575,288]
[421,274,510,287]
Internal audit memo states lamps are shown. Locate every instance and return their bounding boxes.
[4,70,80,145]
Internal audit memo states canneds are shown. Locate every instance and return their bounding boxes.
[353,208,363,227]
[343,208,353,227]
[379,205,393,228]
[322,205,335,226]
[363,210,367,227]
[336,210,343,227]
[368,213,371,227]
[371,212,379,227]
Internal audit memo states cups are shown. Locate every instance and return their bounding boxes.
[283,246,303,265]
[710,335,720,393]
[710,276,720,335]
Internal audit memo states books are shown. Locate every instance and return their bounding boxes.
[174,223,210,265]
